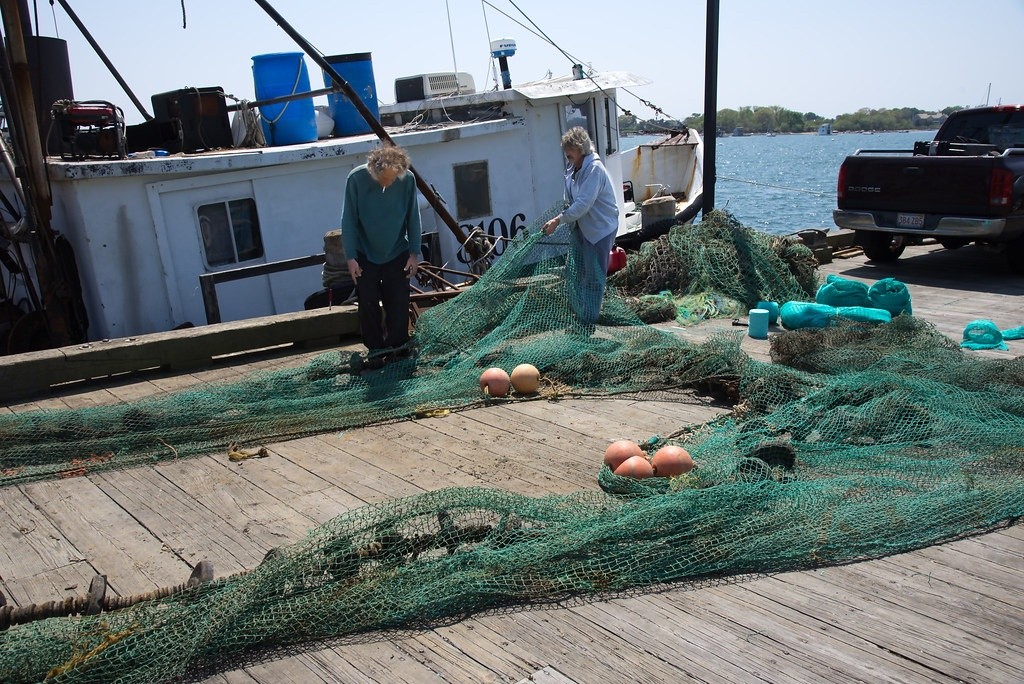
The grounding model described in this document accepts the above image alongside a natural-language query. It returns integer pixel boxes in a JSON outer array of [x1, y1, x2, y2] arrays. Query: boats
[[833, 128, 911, 136], [716, 126, 726, 137], [0, 0, 707, 389], [733, 127, 743, 136], [766, 133, 776, 137]]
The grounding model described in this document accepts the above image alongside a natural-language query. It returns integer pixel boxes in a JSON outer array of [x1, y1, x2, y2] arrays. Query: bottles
[[127, 151, 154, 158], [155, 150, 170, 156]]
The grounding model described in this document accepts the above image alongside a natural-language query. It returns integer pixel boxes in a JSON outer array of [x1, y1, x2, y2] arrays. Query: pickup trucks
[[832, 107, 1024, 281]]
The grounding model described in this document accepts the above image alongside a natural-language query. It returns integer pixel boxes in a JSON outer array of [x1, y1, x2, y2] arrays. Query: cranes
[[997, 97, 1002, 106], [982, 82, 991, 106]]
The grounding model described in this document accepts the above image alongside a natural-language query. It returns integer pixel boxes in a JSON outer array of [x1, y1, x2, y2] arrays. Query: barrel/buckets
[[250, 53, 319, 146], [321, 51, 382, 135]]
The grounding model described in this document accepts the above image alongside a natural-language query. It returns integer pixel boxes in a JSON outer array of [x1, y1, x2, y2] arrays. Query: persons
[[540, 125, 619, 342], [341, 146, 422, 364]]
